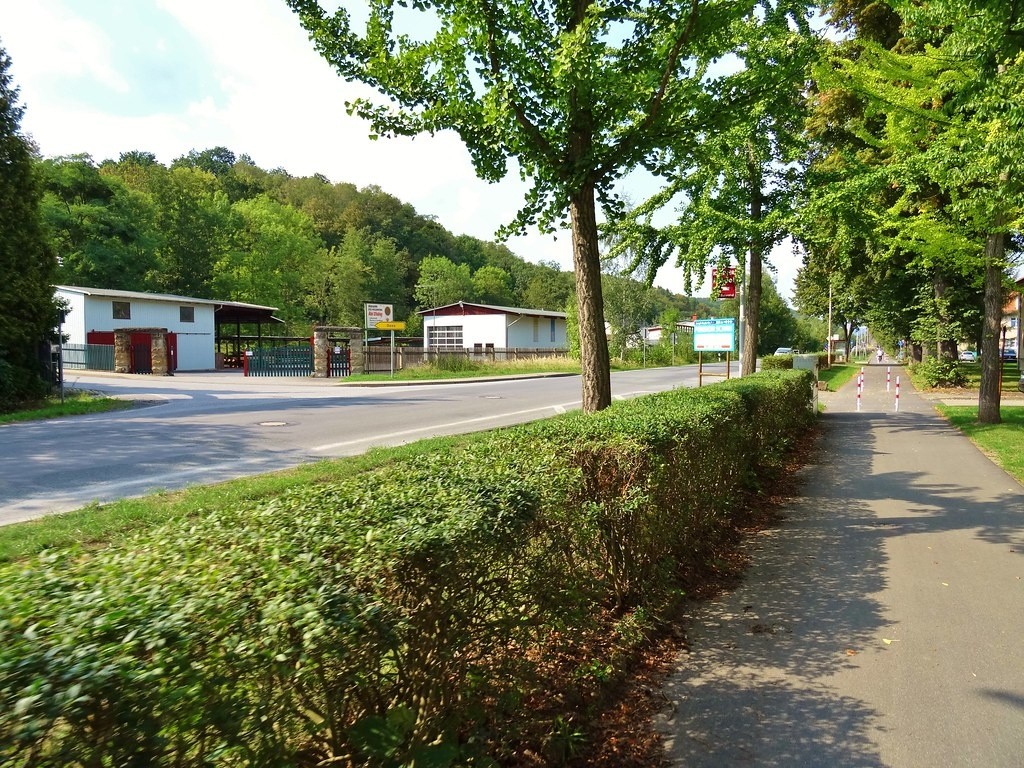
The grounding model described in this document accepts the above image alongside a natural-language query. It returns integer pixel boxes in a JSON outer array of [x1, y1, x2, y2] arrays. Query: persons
[[876, 348, 883, 361]]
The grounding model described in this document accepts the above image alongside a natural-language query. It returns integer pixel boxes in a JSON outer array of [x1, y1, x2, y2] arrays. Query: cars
[[773, 347, 791, 356], [999, 348, 1016, 358], [959, 350, 975, 363]]
